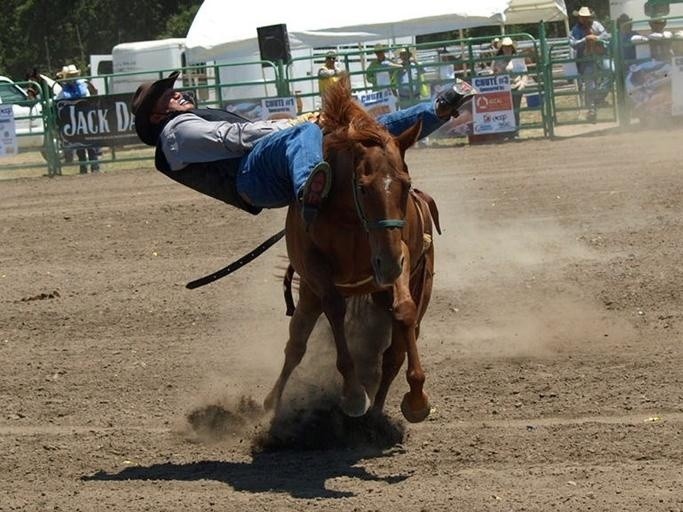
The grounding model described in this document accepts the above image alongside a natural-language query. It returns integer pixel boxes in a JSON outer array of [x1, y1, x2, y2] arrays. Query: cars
[[1, 75, 66, 164]]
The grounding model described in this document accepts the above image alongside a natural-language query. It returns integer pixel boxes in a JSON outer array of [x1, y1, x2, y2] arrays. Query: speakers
[[257, 24, 291, 67]]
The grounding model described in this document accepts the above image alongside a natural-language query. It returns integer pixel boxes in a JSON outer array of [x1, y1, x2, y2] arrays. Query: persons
[[567, 5, 618, 125], [316, 51, 344, 109], [128, 69, 480, 219], [364, 34, 532, 147], [614, 12, 639, 62], [649, 15, 674, 59], [51, 63, 106, 178]]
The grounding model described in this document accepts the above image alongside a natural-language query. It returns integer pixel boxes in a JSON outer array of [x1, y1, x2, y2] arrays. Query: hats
[[497, 37, 517, 48], [57, 64, 81, 76], [572, 7, 592, 17], [395, 48, 413, 56], [372, 44, 388, 52], [130, 71, 180, 145]]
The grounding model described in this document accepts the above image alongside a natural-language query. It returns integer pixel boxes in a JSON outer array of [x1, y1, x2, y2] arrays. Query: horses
[[435, 44, 479, 84], [508, 43, 542, 72], [263, 69, 435, 425]]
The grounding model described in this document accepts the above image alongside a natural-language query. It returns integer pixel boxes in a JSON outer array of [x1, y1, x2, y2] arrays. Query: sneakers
[[439, 77, 476, 107], [301, 162, 333, 226]]
